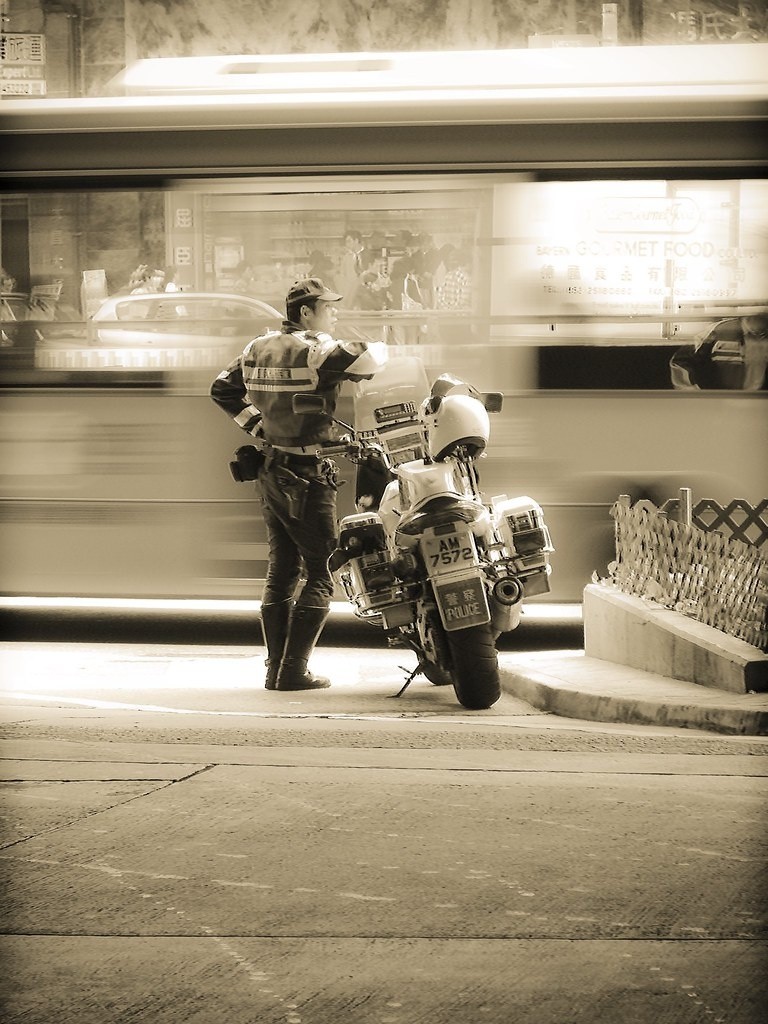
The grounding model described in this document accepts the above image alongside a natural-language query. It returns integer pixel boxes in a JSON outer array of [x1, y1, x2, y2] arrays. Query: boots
[[259, 596, 297, 688], [275, 604, 331, 689]]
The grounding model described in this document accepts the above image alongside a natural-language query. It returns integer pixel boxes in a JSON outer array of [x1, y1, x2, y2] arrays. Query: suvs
[[92, 291, 384, 376]]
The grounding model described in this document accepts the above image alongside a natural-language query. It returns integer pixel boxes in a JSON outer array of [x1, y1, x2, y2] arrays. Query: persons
[[1, 268, 26, 298], [134, 267, 191, 322], [209, 278, 376, 692], [299, 220, 472, 319], [670, 313, 768, 391], [218, 257, 291, 296]]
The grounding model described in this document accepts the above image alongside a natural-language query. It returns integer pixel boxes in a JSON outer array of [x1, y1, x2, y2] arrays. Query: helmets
[[417, 396, 489, 461]]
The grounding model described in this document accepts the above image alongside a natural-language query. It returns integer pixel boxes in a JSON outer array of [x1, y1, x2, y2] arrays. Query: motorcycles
[[291, 345, 554, 710]]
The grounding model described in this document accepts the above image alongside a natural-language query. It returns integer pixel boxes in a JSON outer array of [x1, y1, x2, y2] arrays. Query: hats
[[286, 277, 343, 308]]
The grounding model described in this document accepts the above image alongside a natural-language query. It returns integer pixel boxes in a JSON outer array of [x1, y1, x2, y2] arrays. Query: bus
[[0, 44, 768, 597]]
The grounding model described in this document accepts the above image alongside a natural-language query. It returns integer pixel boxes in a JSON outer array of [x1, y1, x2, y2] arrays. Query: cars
[[0, 293, 102, 367]]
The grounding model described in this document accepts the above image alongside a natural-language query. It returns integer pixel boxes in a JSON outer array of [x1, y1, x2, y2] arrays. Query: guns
[[273, 465, 311, 521]]
